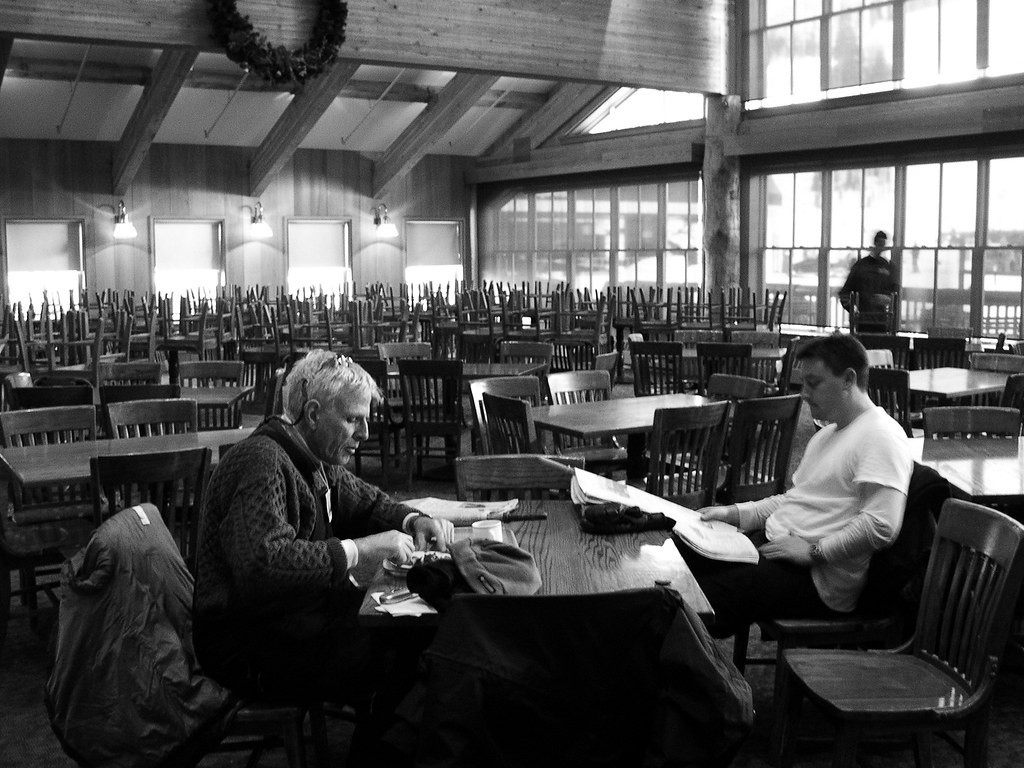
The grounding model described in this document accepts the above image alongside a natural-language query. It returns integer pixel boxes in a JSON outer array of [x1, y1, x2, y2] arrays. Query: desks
[[385, 363, 548, 378], [908, 437, 1024, 505], [92, 385, 255, 406], [906, 368, 1010, 406], [354, 499, 716, 630], [533, 392, 736, 480], [633, 346, 788, 379], [1, 428, 255, 486]]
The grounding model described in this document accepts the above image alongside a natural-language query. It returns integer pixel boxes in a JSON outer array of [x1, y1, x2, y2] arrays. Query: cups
[[471, 519, 502, 543]]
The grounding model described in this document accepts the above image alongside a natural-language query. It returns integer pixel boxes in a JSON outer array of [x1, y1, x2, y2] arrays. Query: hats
[[448, 538, 543, 595]]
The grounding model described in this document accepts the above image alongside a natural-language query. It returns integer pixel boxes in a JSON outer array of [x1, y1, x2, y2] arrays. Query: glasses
[[315, 354, 353, 375]]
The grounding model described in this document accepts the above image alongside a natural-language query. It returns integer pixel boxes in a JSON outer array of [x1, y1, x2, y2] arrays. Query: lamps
[[111, 198, 399, 240]]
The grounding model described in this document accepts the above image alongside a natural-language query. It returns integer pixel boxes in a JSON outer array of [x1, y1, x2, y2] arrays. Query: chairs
[[1, 279, 1024, 767]]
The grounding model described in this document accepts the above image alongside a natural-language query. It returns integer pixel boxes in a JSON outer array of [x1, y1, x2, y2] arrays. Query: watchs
[[808, 544, 825, 563]]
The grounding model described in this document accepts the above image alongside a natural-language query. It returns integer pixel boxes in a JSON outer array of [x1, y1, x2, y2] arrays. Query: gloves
[[578, 503, 676, 535]]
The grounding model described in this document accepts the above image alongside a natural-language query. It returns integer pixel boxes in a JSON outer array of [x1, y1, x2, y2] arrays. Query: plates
[[382, 550, 451, 577]]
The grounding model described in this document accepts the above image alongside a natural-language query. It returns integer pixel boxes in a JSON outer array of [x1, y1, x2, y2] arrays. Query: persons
[[191, 347, 454, 767], [837, 230, 899, 332], [673, 335, 914, 641]]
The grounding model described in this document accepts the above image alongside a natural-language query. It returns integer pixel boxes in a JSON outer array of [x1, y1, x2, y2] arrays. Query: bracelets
[[407, 512, 433, 538]]
